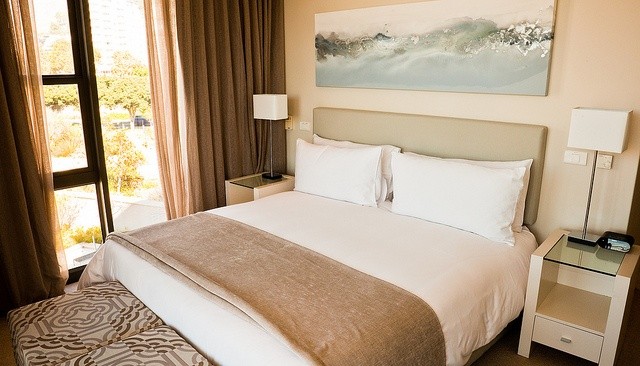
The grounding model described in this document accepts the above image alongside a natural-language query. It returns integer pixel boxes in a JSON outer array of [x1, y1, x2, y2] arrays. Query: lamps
[[252, 91, 289, 178], [566, 107, 632, 248]]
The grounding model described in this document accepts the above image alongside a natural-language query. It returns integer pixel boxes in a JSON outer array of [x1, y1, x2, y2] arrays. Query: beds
[[77, 108, 549, 366]]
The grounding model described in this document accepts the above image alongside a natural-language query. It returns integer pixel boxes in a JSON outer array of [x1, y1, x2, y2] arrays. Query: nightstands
[[517, 226, 640, 366], [225, 172, 294, 208]]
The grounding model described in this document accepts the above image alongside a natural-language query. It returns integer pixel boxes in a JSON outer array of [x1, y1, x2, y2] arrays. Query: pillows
[[294, 138, 385, 209], [401, 150, 533, 233], [312, 134, 401, 202], [389, 151, 526, 247]]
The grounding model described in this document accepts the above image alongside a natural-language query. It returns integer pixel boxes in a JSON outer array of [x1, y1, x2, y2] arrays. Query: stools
[[6, 282, 164, 366], [58, 325, 214, 366]]
